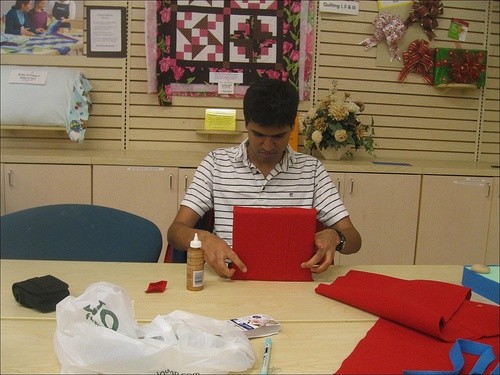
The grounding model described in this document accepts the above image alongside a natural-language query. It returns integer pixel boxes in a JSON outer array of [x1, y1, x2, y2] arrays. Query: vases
[[317, 146, 349, 161]]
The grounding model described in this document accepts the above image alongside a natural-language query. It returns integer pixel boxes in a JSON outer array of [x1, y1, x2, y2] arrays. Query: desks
[[0, 258, 500, 375]]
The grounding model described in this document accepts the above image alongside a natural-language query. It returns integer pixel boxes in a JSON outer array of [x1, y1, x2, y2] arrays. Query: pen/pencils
[[262, 338, 272, 372]]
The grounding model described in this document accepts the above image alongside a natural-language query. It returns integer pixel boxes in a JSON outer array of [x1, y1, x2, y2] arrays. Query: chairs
[[164, 206, 331, 266], [0, 204, 162, 262]]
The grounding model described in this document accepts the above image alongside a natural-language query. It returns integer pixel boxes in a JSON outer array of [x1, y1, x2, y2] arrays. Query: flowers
[[300, 79, 379, 164]]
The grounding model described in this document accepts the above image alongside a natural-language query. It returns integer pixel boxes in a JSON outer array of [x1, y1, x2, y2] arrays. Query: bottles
[[186, 233, 204, 291]]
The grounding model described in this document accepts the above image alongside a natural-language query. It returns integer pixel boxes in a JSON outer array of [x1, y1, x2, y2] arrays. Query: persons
[[5, 0, 45, 36], [27, 0, 49, 33], [48, 0, 76, 30], [167, 79, 362, 278]]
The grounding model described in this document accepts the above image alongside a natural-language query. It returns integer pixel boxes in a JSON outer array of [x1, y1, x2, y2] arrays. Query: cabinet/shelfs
[[0, 162, 91, 216], [320, 171, 421, 266], [415, 174, 500, 264], [93, 165, 198, 262]]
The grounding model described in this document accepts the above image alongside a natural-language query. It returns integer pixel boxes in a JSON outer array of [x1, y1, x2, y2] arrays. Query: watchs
[[329, 228, 347, 252]]
